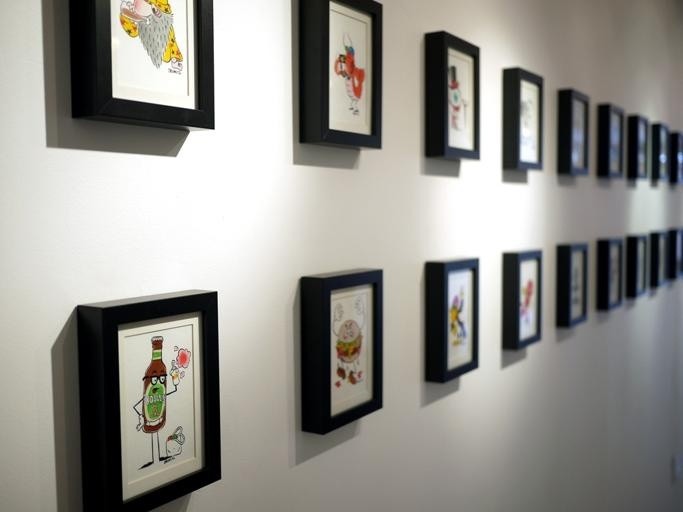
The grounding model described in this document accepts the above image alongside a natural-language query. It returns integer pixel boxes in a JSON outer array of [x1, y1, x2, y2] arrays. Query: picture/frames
[[596, 101, 683, 312], [424, 31, 480, 163], [500, 66, 545, 172], [68, 0, 215, 130], [556, 87, 590, 177], [298, 0, 383, 151], [555, 242, 589, 329], [502, 248, 543, 351], [300, 267, 384, 435], [74, 289, 221, 512], [423, 256, 479, 384]]
